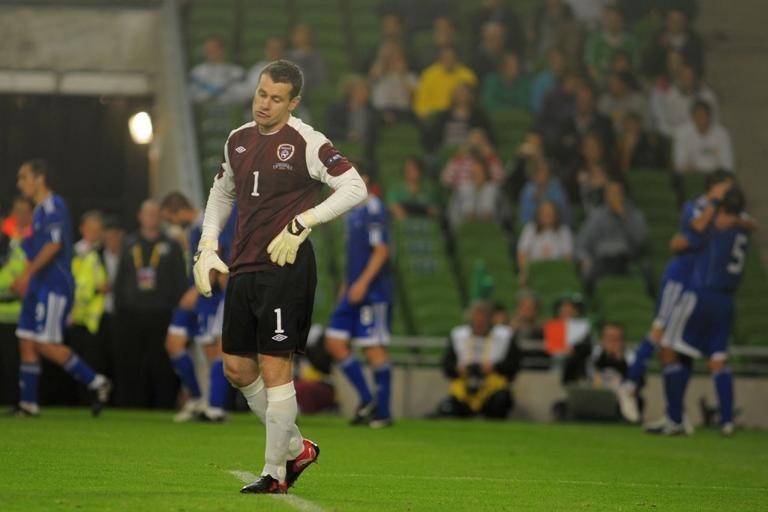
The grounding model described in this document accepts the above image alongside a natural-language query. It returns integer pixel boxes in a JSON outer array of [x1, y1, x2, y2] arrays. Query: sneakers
[[240, 474, 288, 494], [369, 411, 393, 428], [351, 399, 377, 425], [613, 380, 735, 440], [172, 400, 226, 422], [86, 375, 111, 417], [6, 402, 42, 419], [287, 438, 319, 489]]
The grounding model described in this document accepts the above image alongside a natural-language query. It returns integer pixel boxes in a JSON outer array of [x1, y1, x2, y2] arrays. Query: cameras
[[722, 187, 744, 215], [464, 360, 484, 396]]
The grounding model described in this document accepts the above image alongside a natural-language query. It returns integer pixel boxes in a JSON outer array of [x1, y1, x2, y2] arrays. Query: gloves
[[192, 250, 231, 299], [267, 215, 312, 267]]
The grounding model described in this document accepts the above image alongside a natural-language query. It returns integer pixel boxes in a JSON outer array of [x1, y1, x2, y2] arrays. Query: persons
[[325, 0, 735, 298], [429, 297, 645, 425], [189, 26, 330, 109], [615, 169, 758, 438], [191, 58, 371, 496], [322, 162, 394, 428], [0, 158, 229, 422]]
